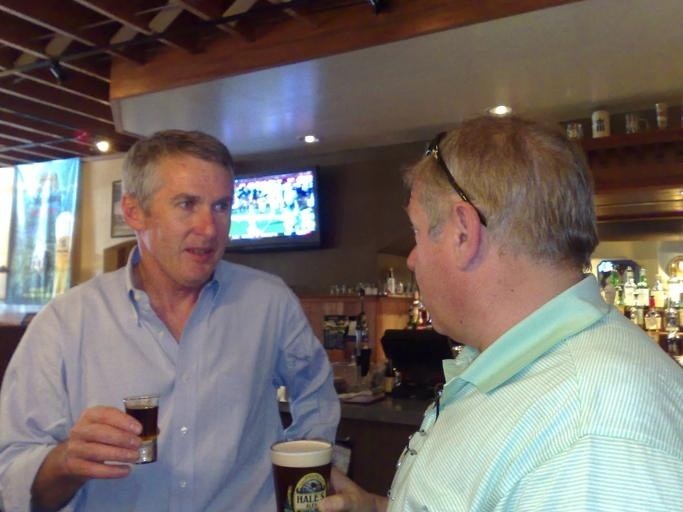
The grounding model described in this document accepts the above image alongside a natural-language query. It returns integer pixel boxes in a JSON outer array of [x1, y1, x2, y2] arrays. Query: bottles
[[381, 357, 396, 399], [408, 290, 423, 329], [386, 267, 395, 296], [591, 105, 610, 139], [565, 122, 584, 141], [604, 267, 682, 354]]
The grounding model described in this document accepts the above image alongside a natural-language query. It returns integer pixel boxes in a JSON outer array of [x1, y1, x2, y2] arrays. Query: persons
[[318, 116, 683, 512], [0, 130, 342, 512]]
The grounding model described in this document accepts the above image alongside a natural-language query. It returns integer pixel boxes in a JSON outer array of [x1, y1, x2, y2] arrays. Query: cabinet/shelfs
[[569, 124, 682, 240]]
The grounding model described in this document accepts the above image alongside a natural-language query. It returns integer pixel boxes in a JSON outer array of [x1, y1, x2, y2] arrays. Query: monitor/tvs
[[381, 328, 456, 394], [225, 164, 330, 253]]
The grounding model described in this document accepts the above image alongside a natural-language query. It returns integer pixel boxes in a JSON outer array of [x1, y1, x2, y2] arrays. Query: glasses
[[426, 131, 488, 227]]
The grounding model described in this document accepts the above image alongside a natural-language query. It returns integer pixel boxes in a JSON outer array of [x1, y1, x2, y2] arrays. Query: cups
[[656, 103, 668, 129], [625, 113, 648, 134], [120, 395, 158, 467], [327, 281, 379, 296], [268, 438, 333, 512], [330, 361, 356, 395]]
